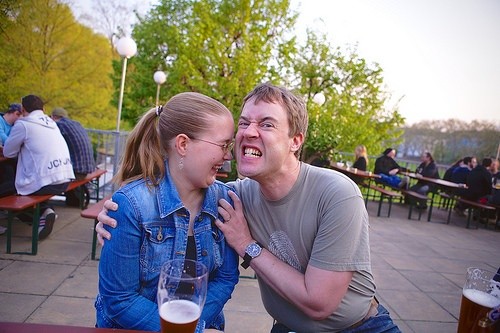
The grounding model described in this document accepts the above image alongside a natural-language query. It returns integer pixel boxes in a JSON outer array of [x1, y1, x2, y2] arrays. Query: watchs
[[240, 240, 266, 270]]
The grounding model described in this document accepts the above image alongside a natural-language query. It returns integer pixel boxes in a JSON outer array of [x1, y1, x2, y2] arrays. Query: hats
[[9, 103, 24, 113], [51, 108, 69, 119]]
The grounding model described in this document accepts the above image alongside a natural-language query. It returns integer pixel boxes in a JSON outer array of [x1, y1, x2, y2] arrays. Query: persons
[[304, 147, 322, 167], [0, 103, 23, 147], [349, 145, 369, 184], [50, 108, 96, 177], [438, 155, 500, 226], [3, 95, 75, 241], [93, 90, 241, 333], [95, 80, 402, 332], [410, 152, 440, 209], [374, 148, 410, 190]]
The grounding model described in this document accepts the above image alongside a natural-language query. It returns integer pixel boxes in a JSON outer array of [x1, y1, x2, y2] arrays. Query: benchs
[[0, 164, 114, 258], [360, 182, 496, 228]]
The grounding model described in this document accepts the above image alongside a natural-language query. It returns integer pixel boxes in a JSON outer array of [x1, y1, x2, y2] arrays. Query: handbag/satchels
[[67, 186, 91, 208]]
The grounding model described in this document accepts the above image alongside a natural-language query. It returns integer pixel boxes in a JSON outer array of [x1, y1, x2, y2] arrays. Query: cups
[[156, 259, 208, 333], [458, 267, 500, 333]]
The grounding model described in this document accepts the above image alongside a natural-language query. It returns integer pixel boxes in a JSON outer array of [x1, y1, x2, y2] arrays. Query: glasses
[[189, 137, 236, 152]]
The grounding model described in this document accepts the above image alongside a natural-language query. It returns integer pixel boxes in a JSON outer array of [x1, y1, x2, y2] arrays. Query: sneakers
[[37, 209, 55, 239]]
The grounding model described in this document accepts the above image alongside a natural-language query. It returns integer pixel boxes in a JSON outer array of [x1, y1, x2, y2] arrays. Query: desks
[[400, 170, 470, 222], [331, 162, 381, 187]]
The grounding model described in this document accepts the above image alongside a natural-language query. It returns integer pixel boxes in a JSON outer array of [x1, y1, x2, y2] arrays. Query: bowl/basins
[[337, 163, 344, 167]]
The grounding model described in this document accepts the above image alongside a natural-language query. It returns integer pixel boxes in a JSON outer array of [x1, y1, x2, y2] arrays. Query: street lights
[[153, 69, 168, 109], [114, 35, 139, 134]]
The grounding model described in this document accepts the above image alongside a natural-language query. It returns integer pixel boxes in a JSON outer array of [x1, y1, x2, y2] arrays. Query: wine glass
[[345, 161, 353, 170]]
